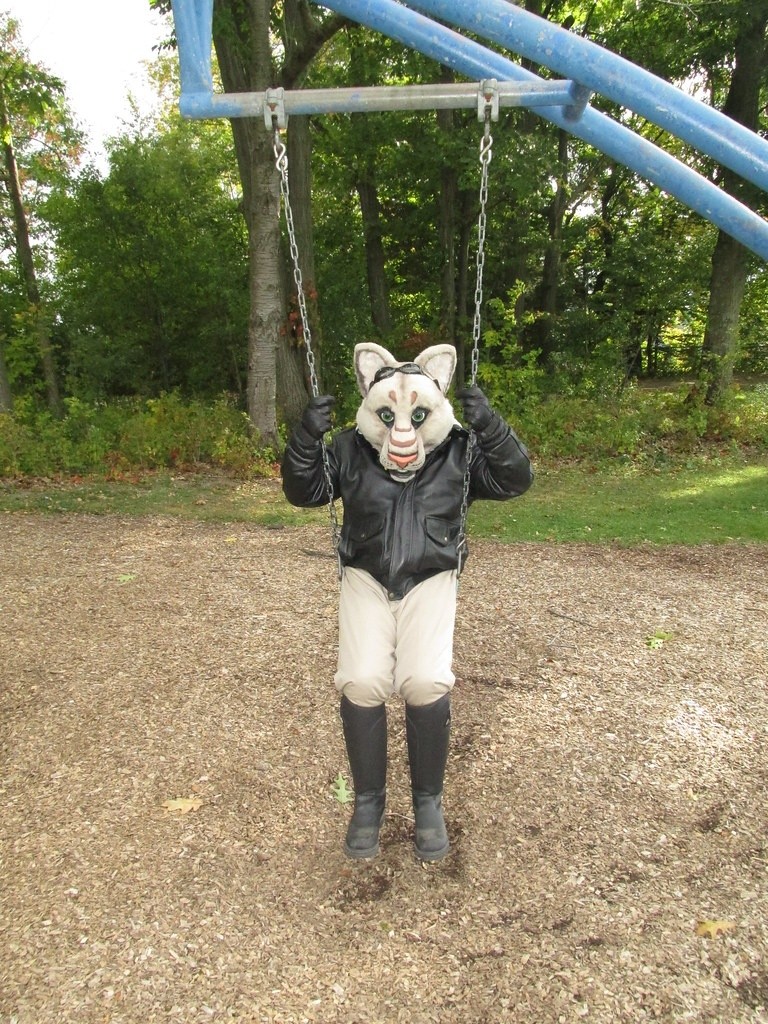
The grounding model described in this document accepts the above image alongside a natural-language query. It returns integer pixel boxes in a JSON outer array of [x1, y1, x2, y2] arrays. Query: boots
[[339, 695, 386, 856], [404, 692, 449, 858]]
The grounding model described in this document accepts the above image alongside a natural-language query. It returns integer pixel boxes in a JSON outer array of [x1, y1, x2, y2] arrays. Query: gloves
[[303, 394, 335, 438], [454, 386, 492, 431]]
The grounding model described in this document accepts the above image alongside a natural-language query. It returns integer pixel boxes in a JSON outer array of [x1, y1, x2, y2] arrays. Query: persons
[[281, 343, 535, 860]]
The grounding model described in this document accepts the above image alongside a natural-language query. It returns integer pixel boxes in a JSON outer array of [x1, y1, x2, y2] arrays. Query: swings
[[259, 82, 505, 580]]
